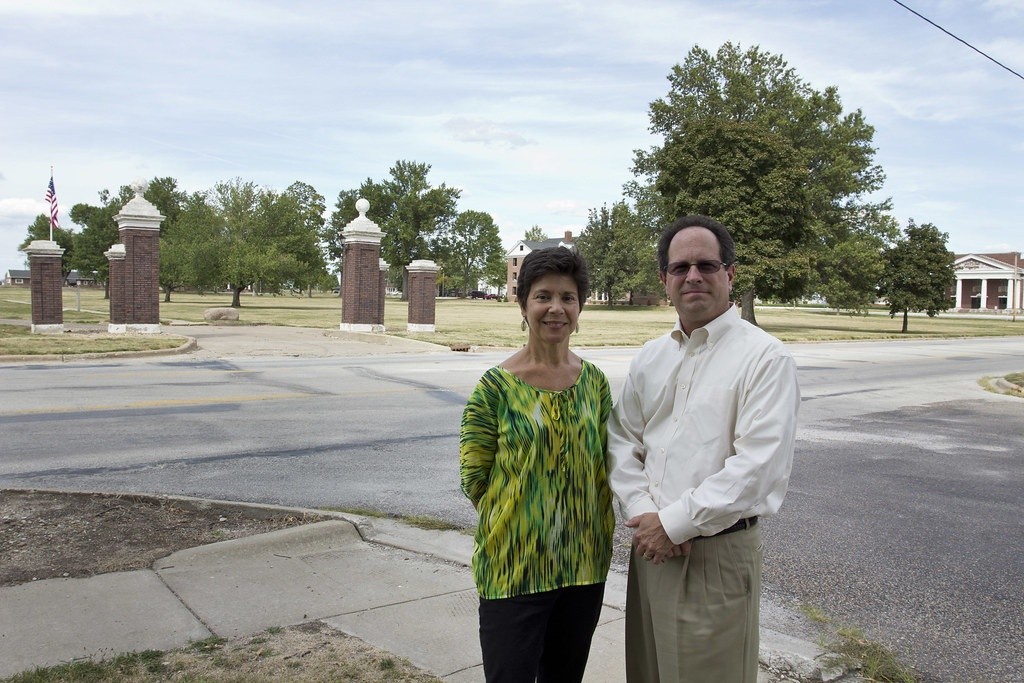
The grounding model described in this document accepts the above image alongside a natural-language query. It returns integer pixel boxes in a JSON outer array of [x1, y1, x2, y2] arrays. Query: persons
[[606, 215, 802, 682], [45, 176, 58, 230], [461, 244, 613, 682]]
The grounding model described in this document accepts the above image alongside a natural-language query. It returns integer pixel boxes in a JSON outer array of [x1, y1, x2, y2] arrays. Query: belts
[[693, 514, 758, 543]]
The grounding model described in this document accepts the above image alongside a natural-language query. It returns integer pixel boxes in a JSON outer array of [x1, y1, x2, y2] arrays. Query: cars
[[442, 287, 505, 300]]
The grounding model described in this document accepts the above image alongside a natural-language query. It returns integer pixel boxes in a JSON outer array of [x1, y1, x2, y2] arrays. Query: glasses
[[663, 258, 728, 276]]
